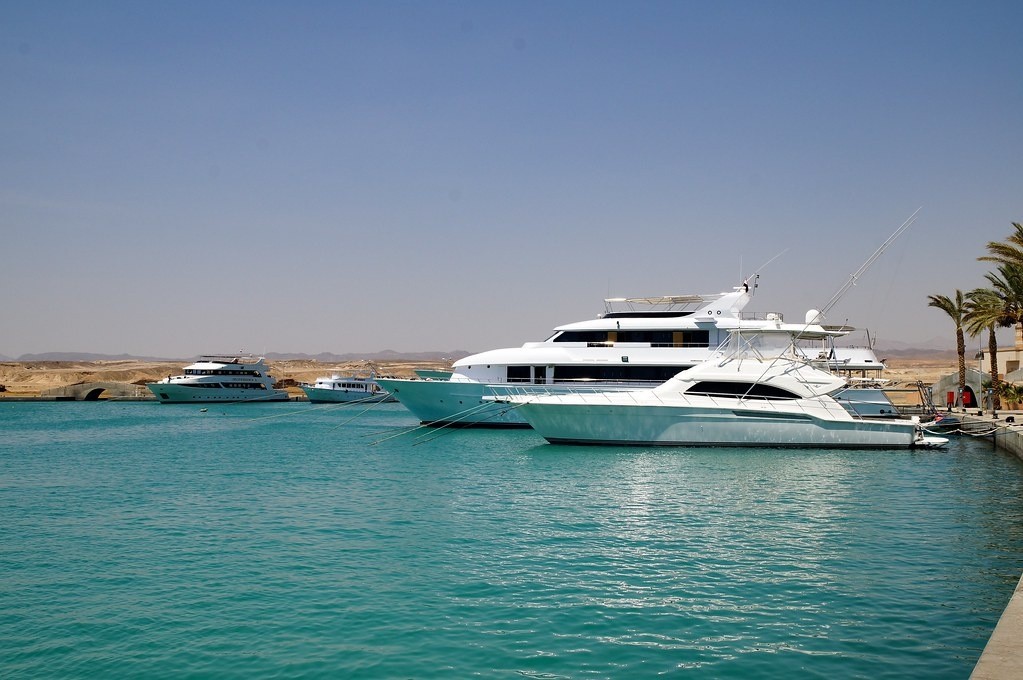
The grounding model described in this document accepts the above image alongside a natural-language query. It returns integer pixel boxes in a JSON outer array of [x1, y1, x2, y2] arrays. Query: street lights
[[442, 357, 451, 371], [278, 361, 288, 391], [361, 360, 371, 377]]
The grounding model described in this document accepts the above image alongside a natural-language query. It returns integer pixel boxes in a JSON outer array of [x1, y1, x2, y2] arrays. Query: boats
[[297, 370, 401, 404], [371, 205, 963, 449], [144, 356, 289, 404]]
